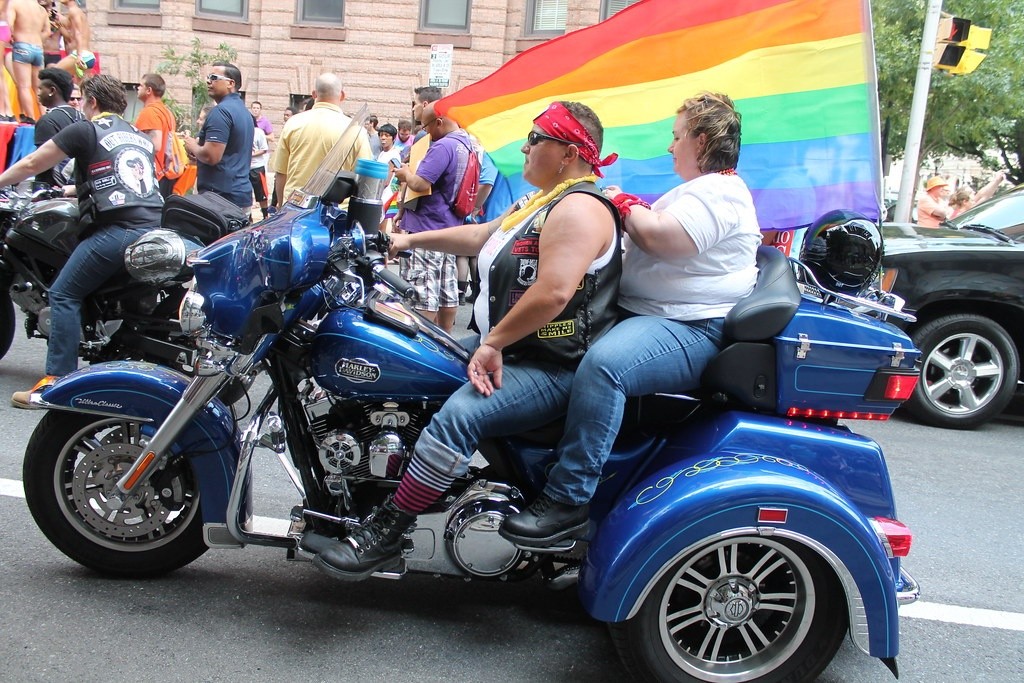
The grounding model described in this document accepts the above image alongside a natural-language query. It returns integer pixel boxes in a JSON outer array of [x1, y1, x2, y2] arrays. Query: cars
[[876, 183, 1024, 431]]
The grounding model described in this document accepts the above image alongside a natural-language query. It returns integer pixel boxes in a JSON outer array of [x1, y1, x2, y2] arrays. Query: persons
[[317, 101, 621, 582], [498, 89, 764, 541], [270, 73, 496, 336], [917, 174, 1006, 229], [0, 0, 95, 123], [0, 62, 273, 409]]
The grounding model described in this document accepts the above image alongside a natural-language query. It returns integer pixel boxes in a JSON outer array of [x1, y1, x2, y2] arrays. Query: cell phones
[[390, 158, 402, 168]]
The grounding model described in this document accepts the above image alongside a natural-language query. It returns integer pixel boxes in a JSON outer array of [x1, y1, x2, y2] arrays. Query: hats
[[925, 177, 947, 193]]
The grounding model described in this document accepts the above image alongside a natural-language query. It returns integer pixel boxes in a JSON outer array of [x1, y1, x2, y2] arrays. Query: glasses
[[206, 74, 233, 82], [527, 131, 583, 147], [422, 117, 442, 132], [68, 96, 82, 102]]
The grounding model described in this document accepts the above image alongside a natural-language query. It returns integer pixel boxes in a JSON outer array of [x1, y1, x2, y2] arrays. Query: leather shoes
[[498, 492, 591, 548]]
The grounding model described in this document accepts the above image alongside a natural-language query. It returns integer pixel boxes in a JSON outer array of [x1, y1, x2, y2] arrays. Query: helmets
[[799, 209, 883, 297]]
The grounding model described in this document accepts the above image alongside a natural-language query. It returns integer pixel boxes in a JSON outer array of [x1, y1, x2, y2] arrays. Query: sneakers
[[12, 377, 61, 409]]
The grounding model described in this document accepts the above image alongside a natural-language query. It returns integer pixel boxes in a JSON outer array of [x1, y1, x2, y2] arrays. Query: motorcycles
[[0, 181, 257, 406], [22, 102, 926, 682]]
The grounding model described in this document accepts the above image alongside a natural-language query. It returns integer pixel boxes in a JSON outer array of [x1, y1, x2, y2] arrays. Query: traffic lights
[[932, 16, 971, 70], [948, 24, 992, 76]]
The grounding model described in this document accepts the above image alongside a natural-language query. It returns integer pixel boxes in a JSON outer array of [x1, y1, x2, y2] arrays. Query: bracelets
[[610, 193, 651, 234]]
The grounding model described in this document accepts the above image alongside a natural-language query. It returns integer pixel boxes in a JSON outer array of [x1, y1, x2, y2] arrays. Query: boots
[[313, 494, 418, 582]]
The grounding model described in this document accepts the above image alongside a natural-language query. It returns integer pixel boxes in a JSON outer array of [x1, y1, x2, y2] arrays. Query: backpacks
[[147, 104, 188, 179], [439, 133, 480, 218]]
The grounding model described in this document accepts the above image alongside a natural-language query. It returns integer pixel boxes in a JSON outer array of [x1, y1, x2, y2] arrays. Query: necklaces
[[716, 167, 737, 176], [500, 174, 597, 231]]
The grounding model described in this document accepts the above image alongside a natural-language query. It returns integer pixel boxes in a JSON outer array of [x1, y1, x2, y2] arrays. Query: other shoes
[[0, 112, 37, 127]]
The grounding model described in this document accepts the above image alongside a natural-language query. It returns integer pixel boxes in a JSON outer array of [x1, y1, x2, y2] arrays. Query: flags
[[427, 0, 884, 232]]
